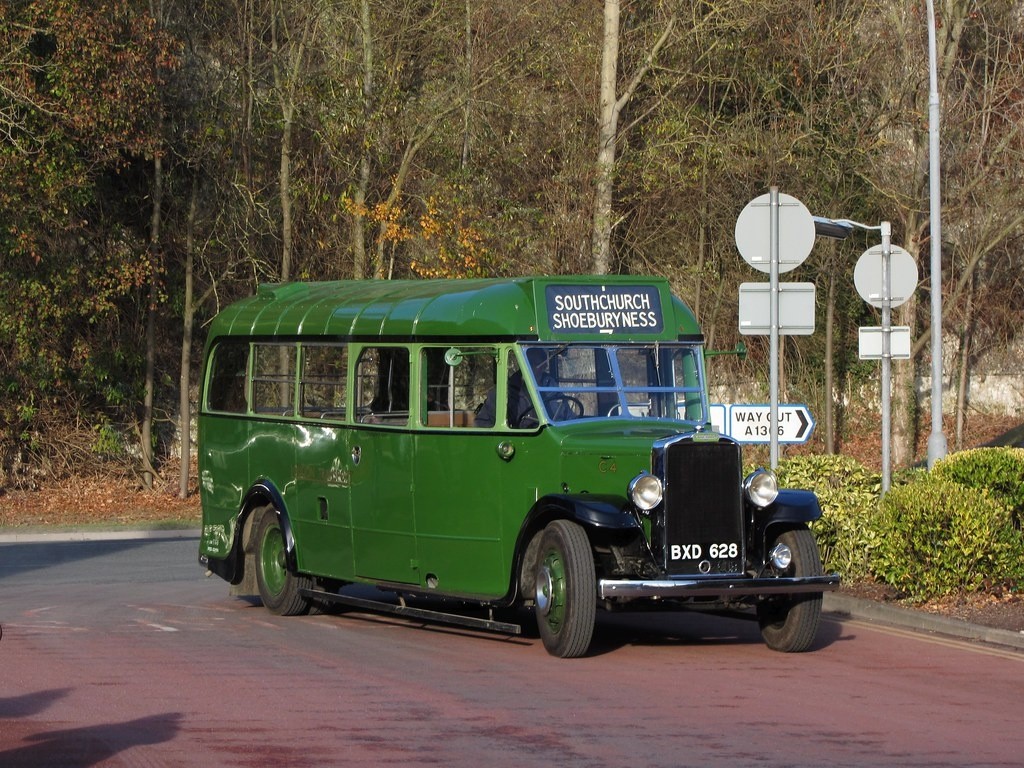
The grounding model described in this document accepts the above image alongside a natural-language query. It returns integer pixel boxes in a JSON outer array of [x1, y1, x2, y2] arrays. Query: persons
[[473, 349, 579, 428]]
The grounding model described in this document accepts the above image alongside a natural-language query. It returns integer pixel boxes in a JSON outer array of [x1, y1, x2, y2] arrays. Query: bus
[[197, 275, 843, 659]]
